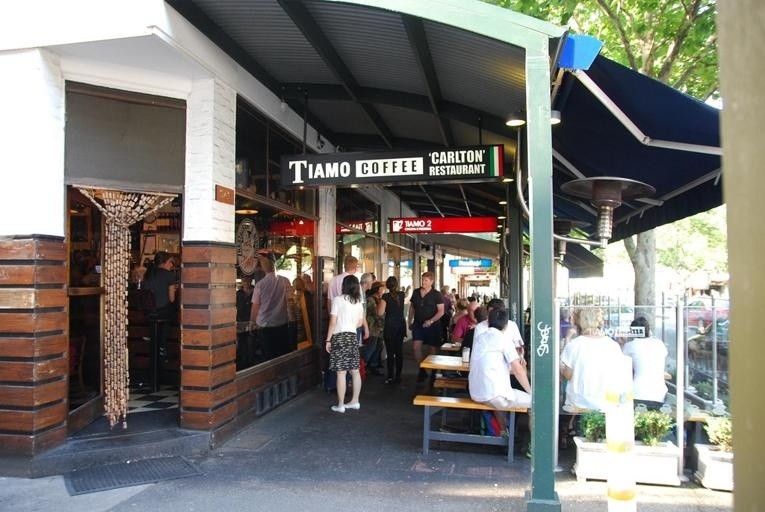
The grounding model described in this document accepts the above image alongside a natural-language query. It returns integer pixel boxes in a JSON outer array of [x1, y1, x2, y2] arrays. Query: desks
[[439, 342, 462, 351], [419, 353, 528, 377]]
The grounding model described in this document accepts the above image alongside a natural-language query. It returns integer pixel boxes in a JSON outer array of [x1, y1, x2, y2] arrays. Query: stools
[[144, 315, 173, 394]]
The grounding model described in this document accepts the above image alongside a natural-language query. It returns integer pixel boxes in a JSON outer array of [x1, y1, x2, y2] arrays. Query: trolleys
[[321, 328, 367, 393]]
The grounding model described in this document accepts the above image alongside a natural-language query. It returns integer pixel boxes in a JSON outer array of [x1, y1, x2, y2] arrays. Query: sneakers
[[331, 403, 360, 413]]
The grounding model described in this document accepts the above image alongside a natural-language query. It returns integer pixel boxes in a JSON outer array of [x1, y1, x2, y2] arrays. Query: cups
[[462, 347, 471, 362]]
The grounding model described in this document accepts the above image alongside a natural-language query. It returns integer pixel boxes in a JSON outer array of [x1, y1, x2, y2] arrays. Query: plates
[[455, 342, 462, 346]]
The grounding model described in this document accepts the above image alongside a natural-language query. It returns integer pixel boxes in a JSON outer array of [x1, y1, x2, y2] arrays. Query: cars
[[688, 312, 729, 398], [603, 299, 634, 321], [684, 298, 729, 337]]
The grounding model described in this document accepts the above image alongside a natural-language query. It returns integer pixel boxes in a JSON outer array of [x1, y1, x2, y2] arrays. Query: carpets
[[59, 452, 208, 499]]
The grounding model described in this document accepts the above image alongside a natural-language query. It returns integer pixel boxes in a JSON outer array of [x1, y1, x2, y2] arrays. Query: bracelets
[[324, 339, 334, 344]]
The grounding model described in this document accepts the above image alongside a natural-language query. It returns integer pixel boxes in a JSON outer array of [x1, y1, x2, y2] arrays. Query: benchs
[[560, 406, 708, 470], [412, 394, 528, 465], [433, 378, 481, 434]]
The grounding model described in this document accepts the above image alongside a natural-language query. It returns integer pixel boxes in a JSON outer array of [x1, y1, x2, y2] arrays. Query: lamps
[[506, 110, 525, 128], [550, 109, 561, 126]]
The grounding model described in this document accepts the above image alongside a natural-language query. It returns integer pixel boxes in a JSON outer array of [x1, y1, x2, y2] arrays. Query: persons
[[467, 307, 533, 459], [559, 303, 627, 415], [327, 255, 527, 412], [68, 244, 319, 393], [622, 317, 668, 410]]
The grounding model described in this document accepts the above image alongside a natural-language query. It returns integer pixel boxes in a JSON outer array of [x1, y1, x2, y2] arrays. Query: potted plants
[[692, 416, 735, 493], [570, 409, 683, 490]]
